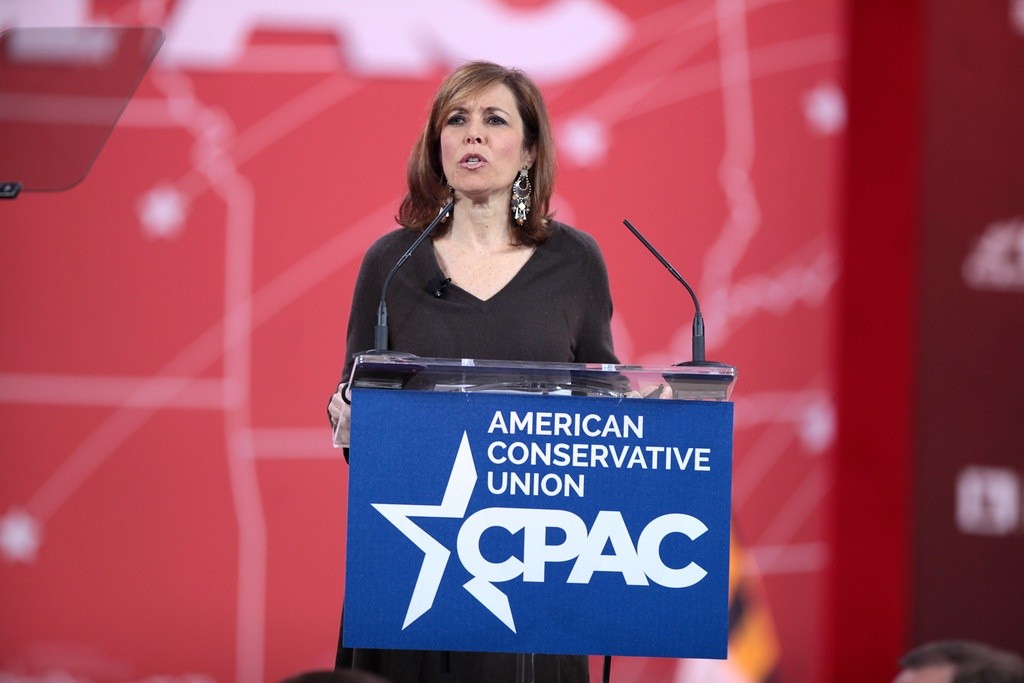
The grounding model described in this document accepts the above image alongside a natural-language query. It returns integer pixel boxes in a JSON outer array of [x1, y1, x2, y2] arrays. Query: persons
[[326, 60, 674, 683], [891, 638, 1024, 682]]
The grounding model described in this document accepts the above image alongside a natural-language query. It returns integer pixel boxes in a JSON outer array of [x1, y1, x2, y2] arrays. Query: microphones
[[623, 219, 737, 383], [348, 202, 454, 379], [428, 277, 443, 297]]
